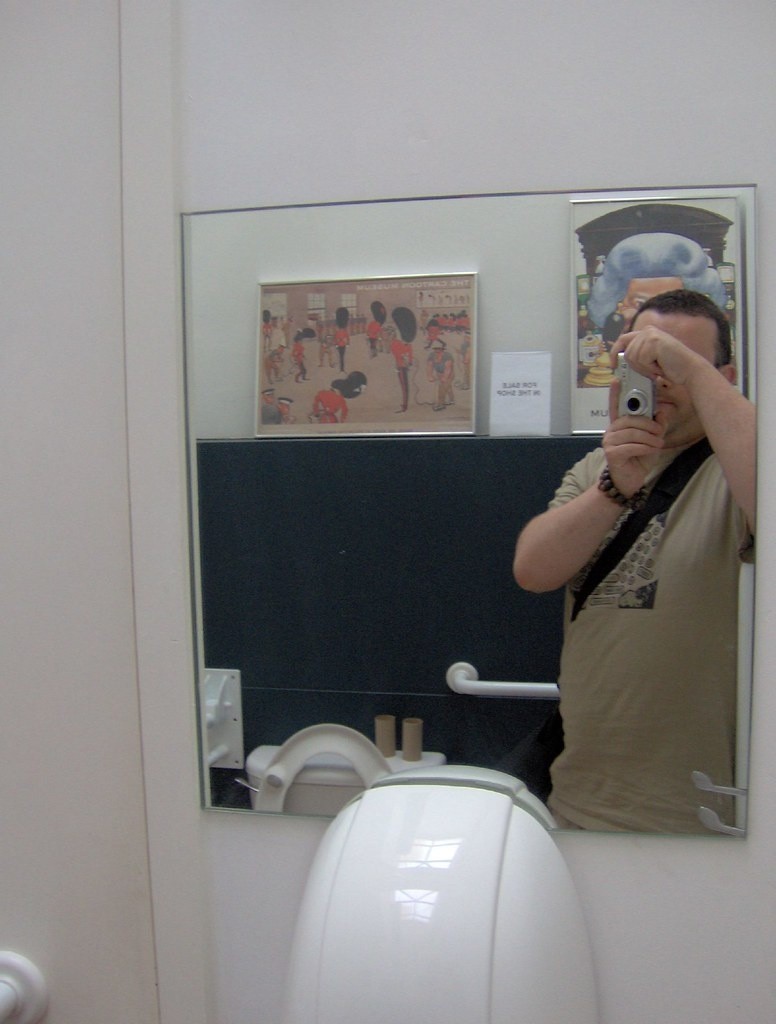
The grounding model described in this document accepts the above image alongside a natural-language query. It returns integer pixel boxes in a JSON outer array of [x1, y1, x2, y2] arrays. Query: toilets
[[247, 724, 446, 813]]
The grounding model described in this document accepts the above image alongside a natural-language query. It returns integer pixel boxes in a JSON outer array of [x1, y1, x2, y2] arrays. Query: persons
[[511, 290, 774, 835]]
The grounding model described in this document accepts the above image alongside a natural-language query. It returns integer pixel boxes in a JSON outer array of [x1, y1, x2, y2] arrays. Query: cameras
[[616, 353, 655, 423]]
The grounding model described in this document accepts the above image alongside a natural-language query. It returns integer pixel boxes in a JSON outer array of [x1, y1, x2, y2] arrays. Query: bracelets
[[598, 464, 649, 509]]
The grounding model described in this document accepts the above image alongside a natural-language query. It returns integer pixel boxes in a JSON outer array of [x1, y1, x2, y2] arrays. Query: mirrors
[[176, 181, 762, 843]]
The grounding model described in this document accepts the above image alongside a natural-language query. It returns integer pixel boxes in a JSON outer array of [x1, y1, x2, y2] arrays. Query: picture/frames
[[568, 196, 742, 435], [254, 272, 478, 438]]
